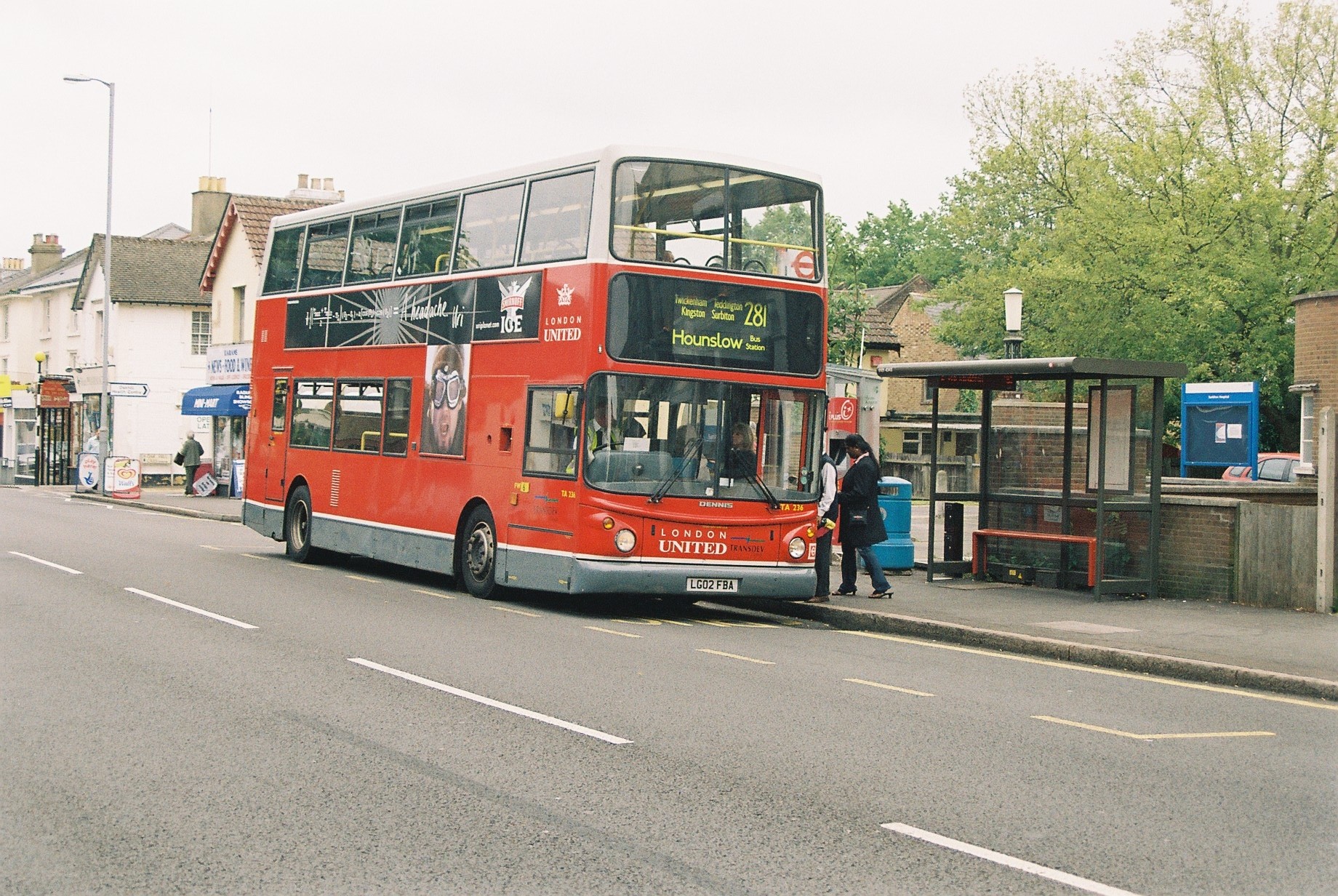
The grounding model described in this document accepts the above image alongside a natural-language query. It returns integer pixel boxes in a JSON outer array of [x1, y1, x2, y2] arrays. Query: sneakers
[[806, 595, 830, 603]]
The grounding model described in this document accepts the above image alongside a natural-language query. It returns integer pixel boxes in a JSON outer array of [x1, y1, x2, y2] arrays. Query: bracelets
[[108, 454, 109, 455]]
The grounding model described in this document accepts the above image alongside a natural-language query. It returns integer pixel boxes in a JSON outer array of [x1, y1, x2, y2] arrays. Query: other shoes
[[183, 493, 195, 497]]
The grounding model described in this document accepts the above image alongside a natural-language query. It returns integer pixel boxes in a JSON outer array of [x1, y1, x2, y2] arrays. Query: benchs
[[972, 528, 1104, 587]]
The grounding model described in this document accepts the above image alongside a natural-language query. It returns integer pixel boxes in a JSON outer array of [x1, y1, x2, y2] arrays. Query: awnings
[[181, 385, 251, 416]]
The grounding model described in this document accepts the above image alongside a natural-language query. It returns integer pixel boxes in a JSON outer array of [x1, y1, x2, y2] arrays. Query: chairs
[[744, 256, 768, 273], [705, 256, 725, 269], [274, 263, 394, 292], [674, 257, 691, 265]]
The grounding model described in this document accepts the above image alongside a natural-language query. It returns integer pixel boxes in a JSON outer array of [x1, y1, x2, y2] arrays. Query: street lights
[[34, 351, 46, 487], [63, 74, 114, 495]]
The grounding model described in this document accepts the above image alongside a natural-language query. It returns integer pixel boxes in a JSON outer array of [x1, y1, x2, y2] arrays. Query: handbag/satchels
[[174, 452, 185, 466], [849, 510, 868, 527]]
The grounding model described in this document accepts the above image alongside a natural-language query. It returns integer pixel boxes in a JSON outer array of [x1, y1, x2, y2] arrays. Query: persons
[[419, 344, 467, 457], [178, 430, 204, 494], [675, 423, 711, 482], [837, 434, 894, 600], [711, 420, 758, 486], [325, 403, 342, 444], [56, 452, 59, 460], [565, 396, 626, 476], [85, 428, 111, 490], [806, 450, 837, 602]]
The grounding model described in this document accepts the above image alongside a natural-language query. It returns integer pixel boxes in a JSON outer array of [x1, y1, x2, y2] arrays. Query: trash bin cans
[[862, 475, 915, 576]]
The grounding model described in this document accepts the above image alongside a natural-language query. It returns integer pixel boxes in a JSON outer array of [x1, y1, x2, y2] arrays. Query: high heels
[[832, 589, 857, 596], [868, 589, 894, 599]]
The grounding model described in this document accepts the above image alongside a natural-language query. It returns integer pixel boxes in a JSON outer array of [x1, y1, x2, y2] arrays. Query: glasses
[[433, 368, 465, 410]]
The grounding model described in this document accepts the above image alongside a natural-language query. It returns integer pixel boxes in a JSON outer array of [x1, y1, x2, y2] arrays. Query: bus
[[237, 151, 830, 606]]
